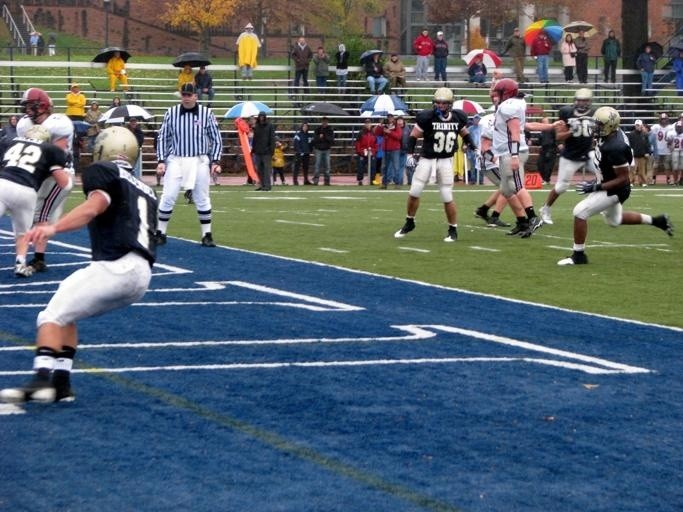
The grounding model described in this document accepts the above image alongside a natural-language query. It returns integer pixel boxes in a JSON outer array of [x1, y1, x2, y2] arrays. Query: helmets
[[591, 105, 621, 137], [659, 112, 683, 130], [24, 124, 51, 144], [489, 78, 519, 101], [91, 125, 141, 171], [431, 87, 454, 104], [19, 87, 51, 114], [573, 87, 594, 106]]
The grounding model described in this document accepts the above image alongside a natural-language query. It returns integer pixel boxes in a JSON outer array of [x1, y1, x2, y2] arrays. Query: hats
[[473, 114, 481, 119], [634, 119, 643, 126], [181, 83, 199, 95], [437, 31, 444, 35]]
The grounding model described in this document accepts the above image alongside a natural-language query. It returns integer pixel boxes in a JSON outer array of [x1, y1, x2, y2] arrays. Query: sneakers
[[504, 216, 544, 239], [443, 225, 458, 242], [242, 181, 400, 191], [0, 379, 57, 404], [630, 178, 683, 188], [155, 230, 167, 246], [538, 206, 554, 225], [557, 250, 589, 266], [52, 383, 76, 403], [394, 217, 417, 239], [656, 214, 675, 238], [201, 231, 217, 247], [416, 78, 447, 81], [14, 259, 33, 278], [473, 207, 512, 228], [27, 259, 50, 272]]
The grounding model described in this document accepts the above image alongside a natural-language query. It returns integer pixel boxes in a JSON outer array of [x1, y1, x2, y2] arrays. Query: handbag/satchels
[[570, 52, 579, 57]]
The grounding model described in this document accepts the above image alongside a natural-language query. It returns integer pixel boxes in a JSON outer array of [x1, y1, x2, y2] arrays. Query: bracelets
[[481, 150, 495, 161], [507, 142, 521, 157]]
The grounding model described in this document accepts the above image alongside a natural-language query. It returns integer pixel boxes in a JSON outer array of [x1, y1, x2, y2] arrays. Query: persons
[[391, 87, 480, 243], [246, 111, 335, 191], [357, 114, 482, 190], [411, 29, 489, 87], [0, 123, 159, 403], [176, 65, 214, 107], [235, 22, 262, 80], [504, 27, 623, 84], [66, 81, 145, 180], [473, 90, 565, 227], [489, 76, 543, 238], [291, 36, 407, 95], [1, 115, 21, 152], [537, 113, 682, 187], [13, 85, 77, 272], [537, 86, 598, 225], [1, 123, 74, 277], [635, 46, 681, 95], [107, 51, 128, 92], [147, 82, 223, 247], [556, 104, 674, 266], [30, 32, 57, 56]]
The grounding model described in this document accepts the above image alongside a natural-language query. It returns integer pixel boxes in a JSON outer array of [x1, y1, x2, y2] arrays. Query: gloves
[[405, 153, 420, 169], [474, 149, 485, 169], [574, 180, 602, 196]]
